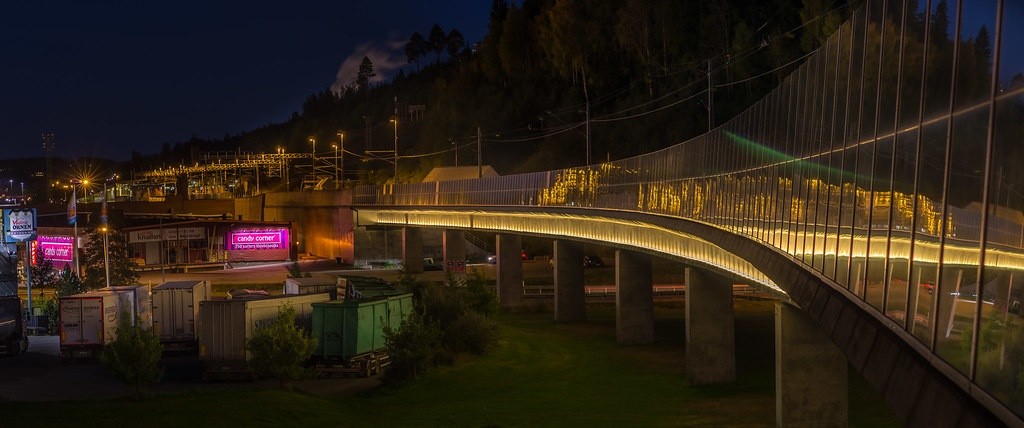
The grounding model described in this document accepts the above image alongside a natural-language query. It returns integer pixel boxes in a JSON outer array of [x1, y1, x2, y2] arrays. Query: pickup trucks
[[421, 258, 442, 270]]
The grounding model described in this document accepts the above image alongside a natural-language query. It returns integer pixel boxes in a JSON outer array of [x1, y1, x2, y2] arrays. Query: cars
[[487, 250, 528, 263], [584, 256, 605, 267]]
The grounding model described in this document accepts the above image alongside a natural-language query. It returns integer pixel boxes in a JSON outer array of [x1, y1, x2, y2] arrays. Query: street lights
[[84, 180, 87, 203], [332, 145, 338, 190], [389, 120, 397, 182], [337, 133, 343, 188], [310, 139, 315, 180]]
